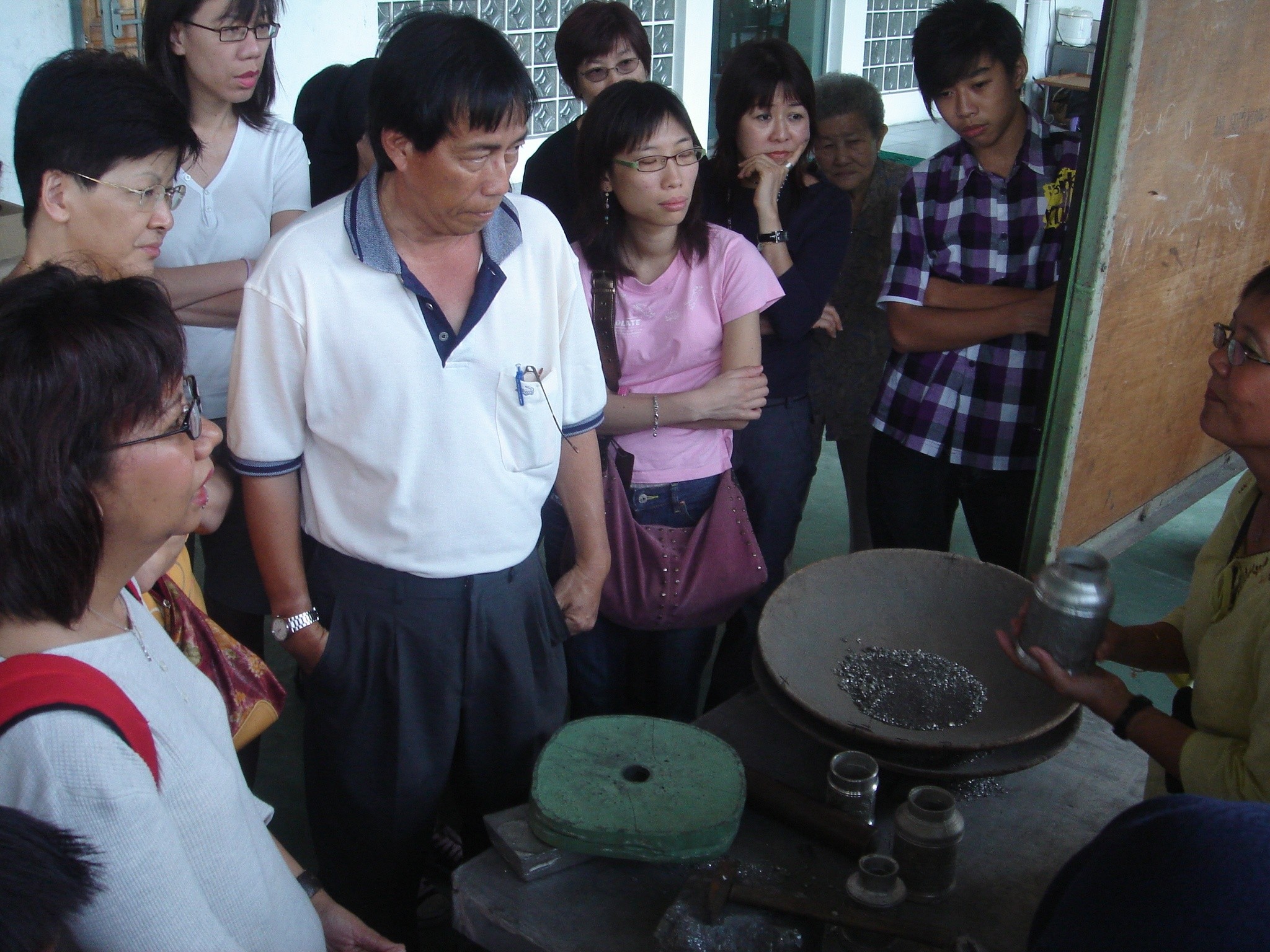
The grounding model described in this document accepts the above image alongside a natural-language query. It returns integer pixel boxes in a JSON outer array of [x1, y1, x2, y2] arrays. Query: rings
[[784, 161, 794, 170]]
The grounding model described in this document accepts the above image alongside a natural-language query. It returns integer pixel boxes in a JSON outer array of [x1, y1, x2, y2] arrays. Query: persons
[[995, 260, 1270, 952], [0, 0, 786, 649], [802, 72, 912, 552], [866, 0, 1085, 574], [700, 39, 850, 610], [0, 262, 406, 952]]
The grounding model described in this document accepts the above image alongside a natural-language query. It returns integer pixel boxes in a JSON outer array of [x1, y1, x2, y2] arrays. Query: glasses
[[577, 57, 640, 82], [60, 170, 188, 211], [1212, 323, 1270, 366], [609, 145, 706, 173], [185, 21, 281, 42], [99, 375, 203, 452]]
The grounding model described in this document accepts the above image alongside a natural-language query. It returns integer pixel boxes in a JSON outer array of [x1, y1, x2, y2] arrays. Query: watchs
[[272, 607, 320, 642], [757, 229, 788, 244]]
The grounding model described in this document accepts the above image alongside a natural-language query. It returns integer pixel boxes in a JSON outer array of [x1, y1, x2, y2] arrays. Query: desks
[[450, 684, 1149, 952]]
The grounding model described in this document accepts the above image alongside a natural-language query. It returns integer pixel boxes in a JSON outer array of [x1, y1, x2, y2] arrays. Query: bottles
[[1009, 545, 1113, 676], [826, 751, 879, 827], [893, 786, 964, 903], [840, 855, 905, 949]]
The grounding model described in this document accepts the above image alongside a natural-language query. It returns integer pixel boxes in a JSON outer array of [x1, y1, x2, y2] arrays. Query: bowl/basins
[[751, 548, 1082, 776]]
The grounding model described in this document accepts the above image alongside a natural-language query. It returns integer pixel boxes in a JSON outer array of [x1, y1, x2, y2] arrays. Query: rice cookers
[[1056, 6, 1093, 46]]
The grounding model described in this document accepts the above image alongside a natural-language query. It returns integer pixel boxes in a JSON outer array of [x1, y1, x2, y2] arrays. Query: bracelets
[[296, 870, 323, 897], [1112, 695, 1153, 740], [241, 257, 252, 279], [652, 396, 659, 437]]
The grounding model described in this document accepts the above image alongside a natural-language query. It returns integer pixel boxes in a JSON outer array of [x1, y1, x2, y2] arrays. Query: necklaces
[[86, 597, 151, 663], [1257, 522, 1268, 542]]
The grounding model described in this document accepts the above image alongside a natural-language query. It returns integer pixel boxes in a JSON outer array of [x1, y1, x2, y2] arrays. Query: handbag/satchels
[[553, 450, 768, 631], [153, 571, 287, 753]]
